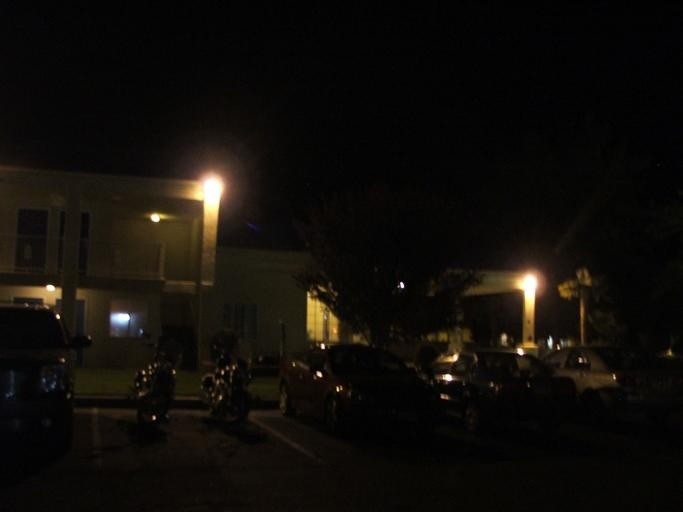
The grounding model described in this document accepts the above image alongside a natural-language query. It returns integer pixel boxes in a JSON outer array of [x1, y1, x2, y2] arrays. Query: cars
[[431, 348, 584, 433], [274, 343, 446, 438], [544, 343, 683, 423]]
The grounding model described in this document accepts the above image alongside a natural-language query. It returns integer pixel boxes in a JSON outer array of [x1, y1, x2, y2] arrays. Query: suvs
[[1, 295, 91, 450]]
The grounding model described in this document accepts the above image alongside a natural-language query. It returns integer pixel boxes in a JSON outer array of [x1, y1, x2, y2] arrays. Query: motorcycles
[[131, 354, 176, 431], [203, 343, 257, 426]]
